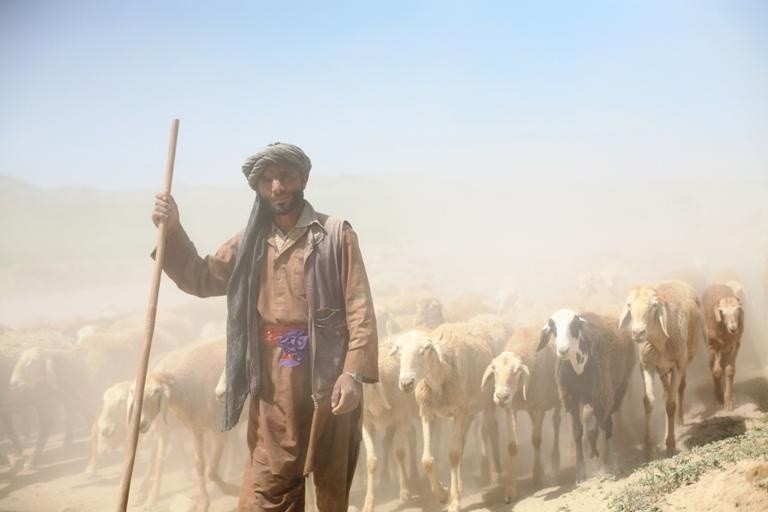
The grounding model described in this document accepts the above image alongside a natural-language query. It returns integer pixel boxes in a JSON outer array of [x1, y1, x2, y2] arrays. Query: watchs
[[344, 371, 364, 383]]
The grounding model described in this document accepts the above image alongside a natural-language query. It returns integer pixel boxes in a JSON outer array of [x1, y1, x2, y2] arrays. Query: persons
[[151, 138, 380, 512]]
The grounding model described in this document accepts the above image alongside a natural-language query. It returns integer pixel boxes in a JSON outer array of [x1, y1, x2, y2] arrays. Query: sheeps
[[0, 316, 246, 512], [361, 291, 556, 508], [536, 277, 700, 483], [703, 279, 748, 409]]
